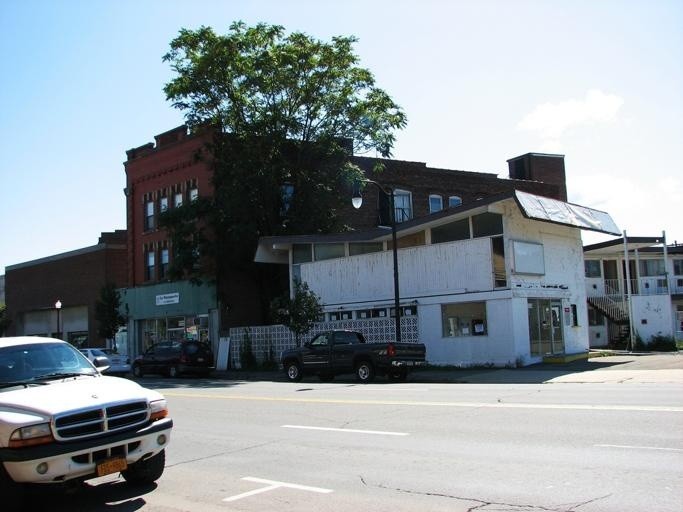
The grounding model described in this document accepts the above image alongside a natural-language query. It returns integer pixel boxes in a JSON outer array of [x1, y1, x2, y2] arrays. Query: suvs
[[131, 338, 215, 376], [0, 336, 173, 492]]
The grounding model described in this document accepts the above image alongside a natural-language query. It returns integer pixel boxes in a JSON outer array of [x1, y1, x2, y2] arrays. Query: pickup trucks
[[278, 327, 427, 384]]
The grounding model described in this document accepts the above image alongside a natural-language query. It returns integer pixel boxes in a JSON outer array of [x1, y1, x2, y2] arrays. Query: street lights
[[350, 176, 402, 347], [54, 299, 62, 338]]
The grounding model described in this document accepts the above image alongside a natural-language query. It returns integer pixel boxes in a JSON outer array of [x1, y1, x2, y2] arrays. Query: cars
[[98, 348, 132, 372], [56, 348, 110, 369]]
